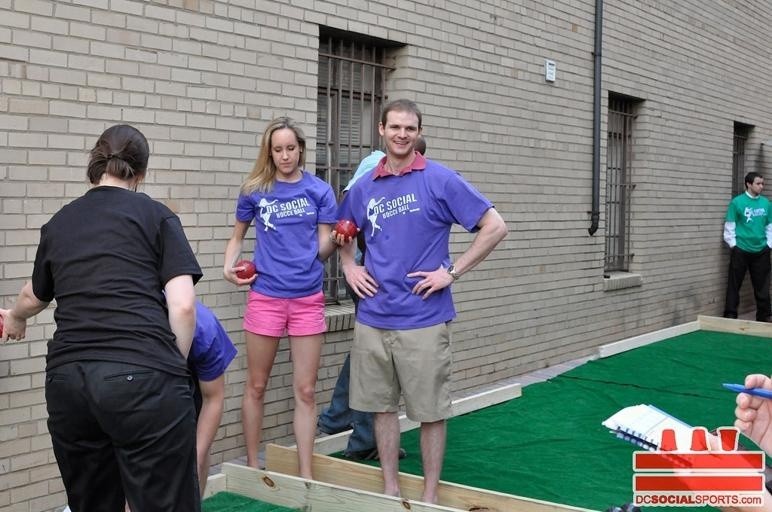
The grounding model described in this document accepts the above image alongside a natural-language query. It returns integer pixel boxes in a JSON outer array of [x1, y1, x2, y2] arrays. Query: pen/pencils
[[719, 383, 772, 399]]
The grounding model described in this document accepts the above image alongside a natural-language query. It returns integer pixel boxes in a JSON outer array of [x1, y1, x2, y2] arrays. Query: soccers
[[335, 220, 357, 242], [237, 260, 256, 280]]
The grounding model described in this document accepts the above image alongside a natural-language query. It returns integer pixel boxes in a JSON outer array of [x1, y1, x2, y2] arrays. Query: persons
[[158, 297, 238, 499], [221, 116, 359, 482], [0, 123, 207, 511], [730, 370, 771, 461], [722, 172, 772, 326], [311, 130, 429, 462], [333, 94, 509, 505]]
